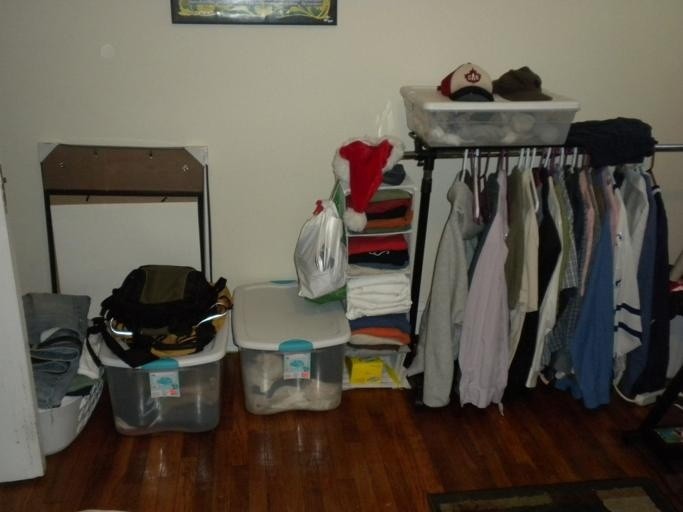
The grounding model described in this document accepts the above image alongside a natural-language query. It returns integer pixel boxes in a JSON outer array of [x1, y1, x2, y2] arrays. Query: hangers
[[442, 149, 668, 224]]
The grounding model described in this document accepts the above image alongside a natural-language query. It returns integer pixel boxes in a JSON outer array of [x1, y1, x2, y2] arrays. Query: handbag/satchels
[[99, 265, 219, 336]]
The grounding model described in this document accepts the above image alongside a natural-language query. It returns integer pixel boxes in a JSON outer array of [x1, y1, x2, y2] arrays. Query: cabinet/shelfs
[[333, 173, 419, 392]]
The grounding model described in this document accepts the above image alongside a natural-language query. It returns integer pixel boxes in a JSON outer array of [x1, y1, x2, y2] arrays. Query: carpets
[[425, 476, 678, 511]]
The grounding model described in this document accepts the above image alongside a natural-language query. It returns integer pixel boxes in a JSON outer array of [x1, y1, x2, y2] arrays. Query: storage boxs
[[400, 85, 579, 149], [99, 281, 352, 436]]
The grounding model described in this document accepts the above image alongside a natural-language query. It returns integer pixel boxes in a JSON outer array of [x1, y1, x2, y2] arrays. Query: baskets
[[38, 377, 103, 456]]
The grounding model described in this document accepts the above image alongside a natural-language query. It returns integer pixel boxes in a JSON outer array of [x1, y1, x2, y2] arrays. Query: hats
[[437, 62, 553, 102]]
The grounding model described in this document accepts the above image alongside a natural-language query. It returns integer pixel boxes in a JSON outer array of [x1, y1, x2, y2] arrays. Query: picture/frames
[[170, 0, 337, 26]]
